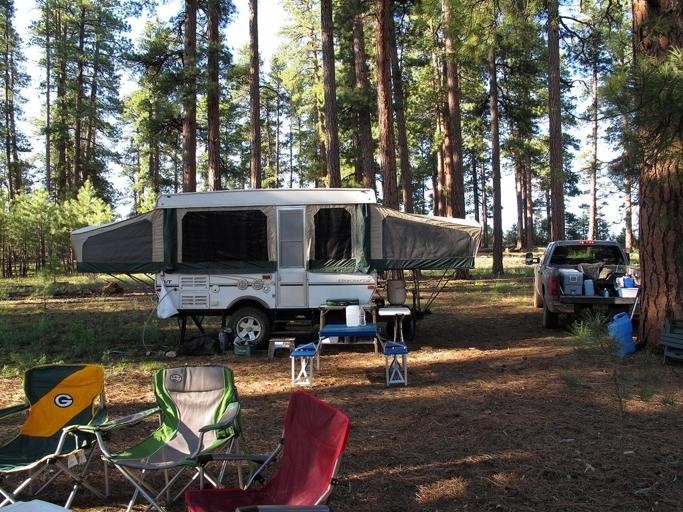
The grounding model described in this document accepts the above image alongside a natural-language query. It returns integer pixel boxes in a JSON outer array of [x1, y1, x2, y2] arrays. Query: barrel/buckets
[[387, 279, 407, 305], [623, 277, 635, 288], [607, 311, 636, 357]]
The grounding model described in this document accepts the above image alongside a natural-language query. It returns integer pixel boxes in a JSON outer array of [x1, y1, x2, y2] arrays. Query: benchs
[[381, 342, 406, 387], [287, 340, 320, 387]]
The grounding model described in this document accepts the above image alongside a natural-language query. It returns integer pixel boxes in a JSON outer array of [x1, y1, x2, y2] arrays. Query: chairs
[[0, 364, 109, 512], [178, 388, 347, 512], [91, 363, 246, 510], [657, 307, 682, 364]]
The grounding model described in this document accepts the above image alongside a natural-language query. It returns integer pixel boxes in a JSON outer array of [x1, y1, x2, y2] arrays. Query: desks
[[379, 305, 409, 341], [320, 325, 376, 352]]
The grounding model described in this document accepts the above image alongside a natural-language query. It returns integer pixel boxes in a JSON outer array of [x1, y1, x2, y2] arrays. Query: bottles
[[359, 306, 366, 326], [604, 288, 609, 298], [218, 329, 228, 351]]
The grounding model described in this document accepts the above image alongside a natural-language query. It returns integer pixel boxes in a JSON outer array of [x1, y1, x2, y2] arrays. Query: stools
[[266, 337, 295, 361]]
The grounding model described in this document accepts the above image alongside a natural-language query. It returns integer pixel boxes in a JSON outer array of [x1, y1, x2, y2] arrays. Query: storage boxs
[[618, 286, 637, 299], [559, 267, 581, 295]]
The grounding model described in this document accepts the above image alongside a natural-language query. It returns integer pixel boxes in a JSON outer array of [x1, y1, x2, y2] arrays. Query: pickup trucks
[[524, 238, 640, 327]]
